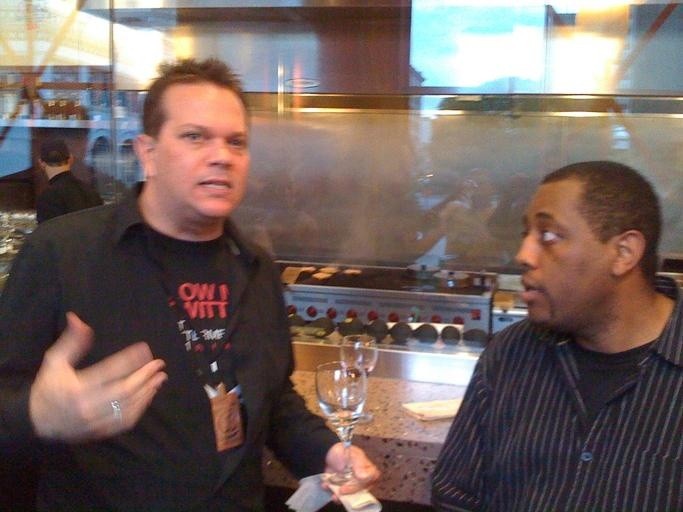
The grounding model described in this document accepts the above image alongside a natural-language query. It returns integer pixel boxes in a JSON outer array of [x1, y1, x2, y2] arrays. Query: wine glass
[[314, 361, 367, 485], [340, 334, 378, 424]]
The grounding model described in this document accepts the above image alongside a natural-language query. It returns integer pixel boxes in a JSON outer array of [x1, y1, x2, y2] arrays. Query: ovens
[[272, 260, 499, 341]]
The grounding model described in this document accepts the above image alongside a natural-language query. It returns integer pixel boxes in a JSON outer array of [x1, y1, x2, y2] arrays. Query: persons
[[34, 137, 105, 227], [425, 159, 683, 511], [0, 59, 382, 512], [1, 96, 127, 210]]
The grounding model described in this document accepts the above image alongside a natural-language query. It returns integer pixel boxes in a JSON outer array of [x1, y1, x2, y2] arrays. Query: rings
[[109, 398, 122, 418]]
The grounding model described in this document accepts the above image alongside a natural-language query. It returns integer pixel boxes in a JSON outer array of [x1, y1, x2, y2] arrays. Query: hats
[[40, 139, 69, 163]]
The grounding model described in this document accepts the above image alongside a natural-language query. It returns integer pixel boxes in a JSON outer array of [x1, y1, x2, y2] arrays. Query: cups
[[0, 210, 37, 255]]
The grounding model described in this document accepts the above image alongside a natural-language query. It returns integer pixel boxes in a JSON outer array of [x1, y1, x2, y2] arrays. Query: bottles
[[1, 84, 137, 122]]
